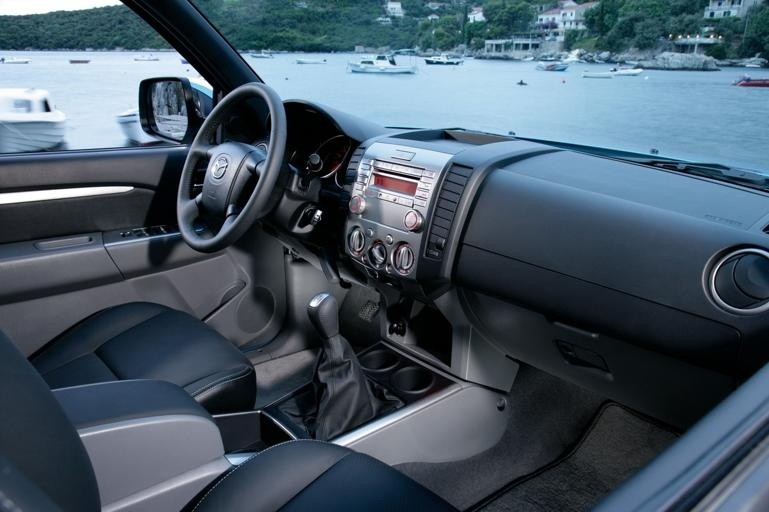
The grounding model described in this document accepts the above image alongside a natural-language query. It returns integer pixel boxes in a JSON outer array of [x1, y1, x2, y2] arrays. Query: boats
[[251, 53, 273, 58], [534, 60, 644, 79], [0, 86, 69, 155], [295, 57, 328, 65], [347, 53, 417, 74], [424, 55, 463, 65], [134, 57, 159, 61], [0, 56, 33, 64], [734, 75, 769, 87], [68, 59, 89, 64], [115, 107, 164, 147]]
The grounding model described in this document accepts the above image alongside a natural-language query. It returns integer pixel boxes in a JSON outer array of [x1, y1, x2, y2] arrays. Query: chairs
[[2, 333, 457, 510], [29, 301, 257, 418]]
